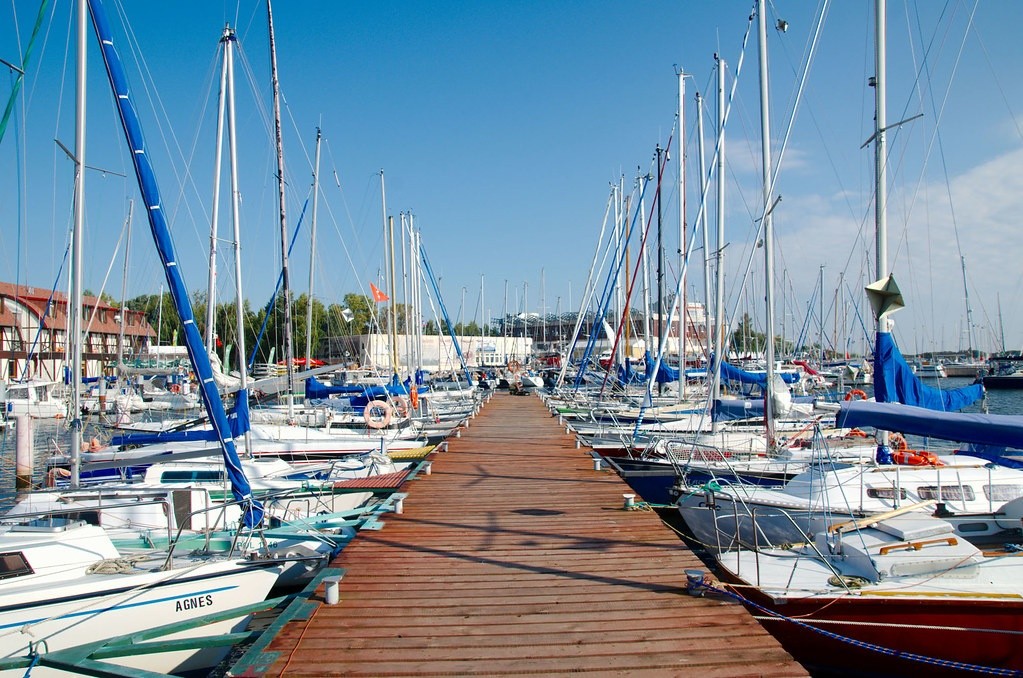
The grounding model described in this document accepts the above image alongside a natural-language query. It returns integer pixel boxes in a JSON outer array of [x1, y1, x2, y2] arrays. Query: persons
[[509, 382, 522, 395], [988, 358, 997, 376], [188, 371, 195, 384], [695, 356, 701, 367]]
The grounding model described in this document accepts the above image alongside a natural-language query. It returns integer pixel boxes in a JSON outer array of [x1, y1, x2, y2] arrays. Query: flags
[[371, 284, 388, 301]]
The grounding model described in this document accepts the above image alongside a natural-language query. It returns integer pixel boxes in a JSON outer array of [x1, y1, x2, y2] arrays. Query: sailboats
[[532, 0, 1023, 561], [0, 0, 491, 676], [432, 273, 545, 390]]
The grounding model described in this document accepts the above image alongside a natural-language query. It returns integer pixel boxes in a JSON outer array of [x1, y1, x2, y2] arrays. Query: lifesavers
[[888, 433, 907, 449], [507, 360, 520, 373], [892, 448, 939, 466], [845, 429, 867, 438], [410, 386, 419, 410], [386, 396, 409, 418], [845, 390, 867, 401], [363, 400, 392, 430]]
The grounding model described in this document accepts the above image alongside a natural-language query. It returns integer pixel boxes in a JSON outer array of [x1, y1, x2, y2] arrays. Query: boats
[[704, 400, 1023, 677]]
